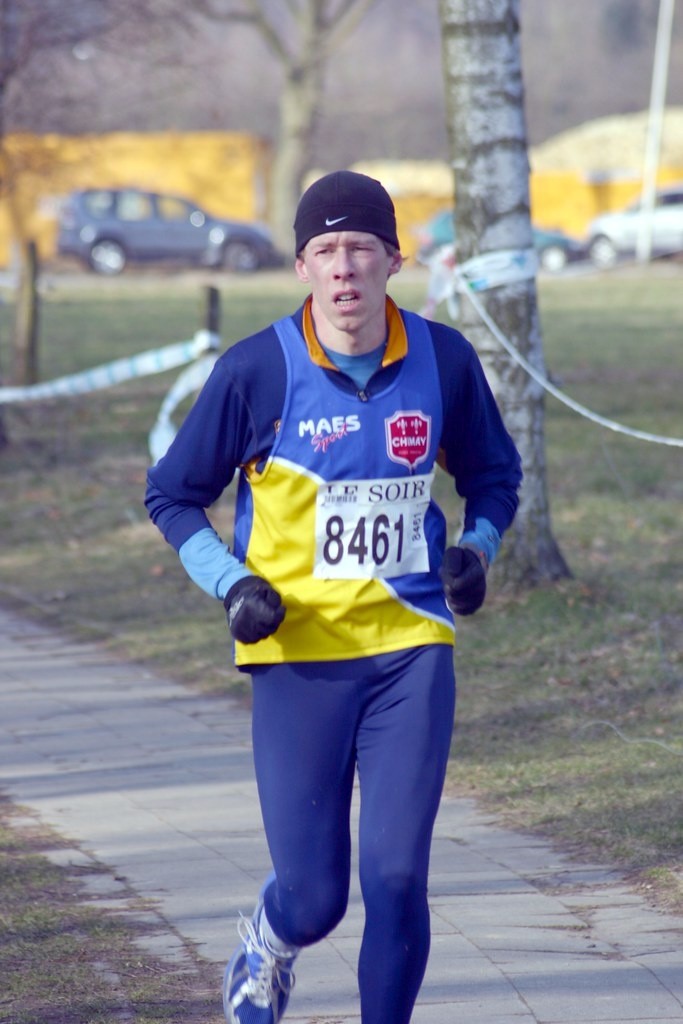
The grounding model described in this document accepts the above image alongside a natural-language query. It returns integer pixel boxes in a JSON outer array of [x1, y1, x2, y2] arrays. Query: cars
[[588, 190, 683, 270], [54, 186, 280, 278], [412, 211, 590, 273]]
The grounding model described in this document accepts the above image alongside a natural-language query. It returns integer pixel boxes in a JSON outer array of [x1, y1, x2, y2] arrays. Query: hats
[[293, 170, 400, 257]]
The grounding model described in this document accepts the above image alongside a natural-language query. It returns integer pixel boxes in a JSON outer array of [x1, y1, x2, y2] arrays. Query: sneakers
[[221, 868, 299, 1024]]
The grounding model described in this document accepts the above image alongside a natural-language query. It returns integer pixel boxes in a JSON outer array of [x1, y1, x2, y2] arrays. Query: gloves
[[438, 546, 487, 616], [222, 575, 287, 644]]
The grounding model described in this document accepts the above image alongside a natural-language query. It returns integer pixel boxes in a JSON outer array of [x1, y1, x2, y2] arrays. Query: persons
[[144, 170, 523, 1024]]
